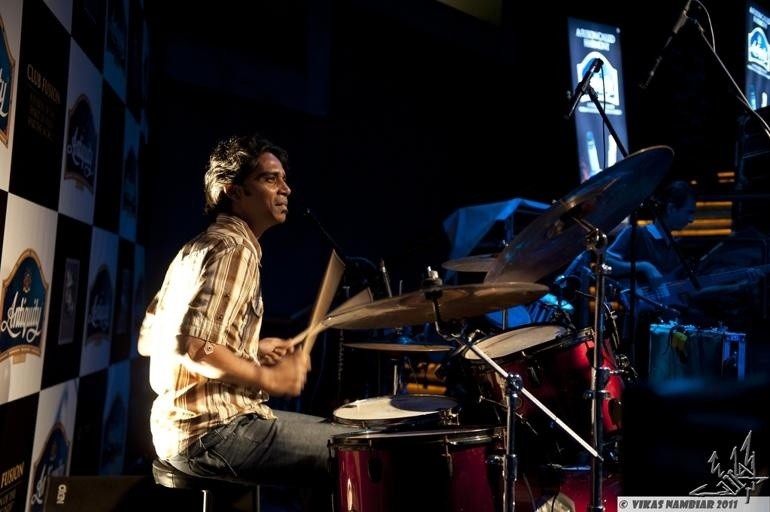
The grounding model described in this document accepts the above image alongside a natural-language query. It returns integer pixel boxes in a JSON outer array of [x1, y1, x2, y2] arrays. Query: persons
[[63, 270, 74, 313], [11, 269, 38, 308], [136, 132, 362, 485], [606, 180, 695, 301]]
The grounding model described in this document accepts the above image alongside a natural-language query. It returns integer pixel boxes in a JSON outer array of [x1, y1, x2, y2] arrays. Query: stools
[[151, 457, 263, 512]]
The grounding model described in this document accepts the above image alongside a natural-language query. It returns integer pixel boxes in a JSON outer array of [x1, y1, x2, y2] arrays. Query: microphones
[[564, 59, 599, 120], [638, 2, 690, 89], [302, 207, 346, 259]]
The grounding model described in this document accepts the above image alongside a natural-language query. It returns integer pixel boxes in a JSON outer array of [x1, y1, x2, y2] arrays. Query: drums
[[334, 395, 456, 421], [326, 428, 506, 511], [464, 324, 622, 450]]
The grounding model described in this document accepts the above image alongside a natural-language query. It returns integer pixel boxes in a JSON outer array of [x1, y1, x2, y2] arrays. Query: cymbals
[[485, 146, 673, 285], [444, 255, 499, 273], [324, 282, 549, 328], [344, 343, 454, 351]]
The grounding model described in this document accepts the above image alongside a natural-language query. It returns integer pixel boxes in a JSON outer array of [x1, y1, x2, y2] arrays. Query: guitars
[[621, 262, 769, 319]]
